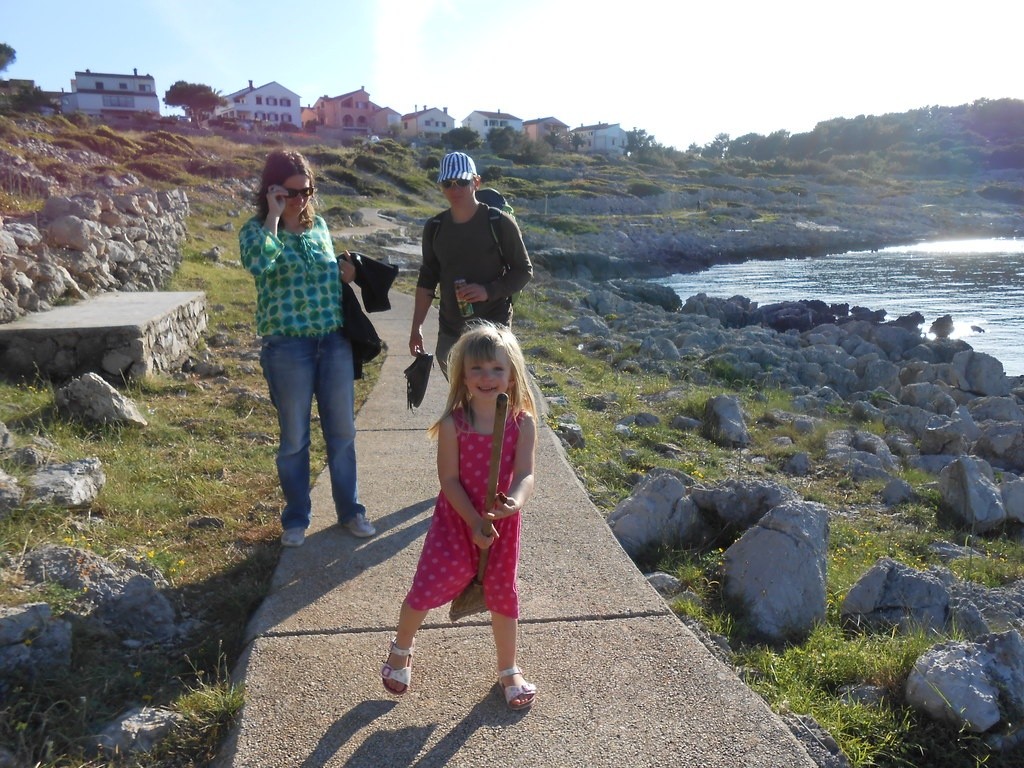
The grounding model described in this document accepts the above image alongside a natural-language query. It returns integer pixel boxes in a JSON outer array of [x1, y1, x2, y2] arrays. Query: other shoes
[[403, 351, 434, 410], [337, 510, 376, 537], [281, 527, 305, 546]]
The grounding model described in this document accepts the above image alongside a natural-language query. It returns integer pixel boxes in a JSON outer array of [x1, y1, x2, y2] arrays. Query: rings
[[271, 188, 276, 191]]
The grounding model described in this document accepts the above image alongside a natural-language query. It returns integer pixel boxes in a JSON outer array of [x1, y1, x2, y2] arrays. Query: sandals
[[380, 636, 415, 694], [498, 667, 536, 710]]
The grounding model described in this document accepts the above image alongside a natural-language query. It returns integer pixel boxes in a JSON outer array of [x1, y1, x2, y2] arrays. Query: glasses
[[442, 180, 470, 189], [279, 184, 313, 198]]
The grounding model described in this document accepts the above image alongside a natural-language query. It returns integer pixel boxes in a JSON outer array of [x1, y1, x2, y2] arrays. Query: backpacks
[[429, 187, 523, 306]]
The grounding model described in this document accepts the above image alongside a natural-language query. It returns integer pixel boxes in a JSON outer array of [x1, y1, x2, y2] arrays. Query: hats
[[437, 152, 477, 183]]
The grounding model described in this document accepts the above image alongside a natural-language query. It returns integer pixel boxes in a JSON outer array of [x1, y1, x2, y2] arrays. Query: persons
[[409, 150, 534, 382], [237, 148, 377, 547], [381, 317, 539, 712]]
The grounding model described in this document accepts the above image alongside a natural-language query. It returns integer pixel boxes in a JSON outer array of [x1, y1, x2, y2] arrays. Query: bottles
[[454, 278, 474, 317]]
